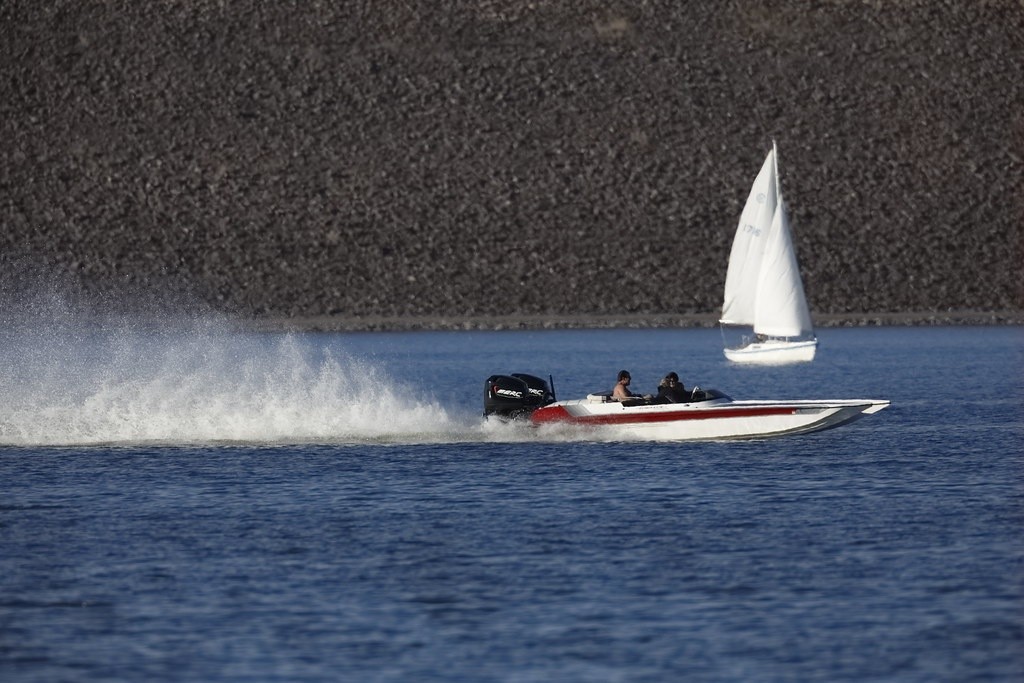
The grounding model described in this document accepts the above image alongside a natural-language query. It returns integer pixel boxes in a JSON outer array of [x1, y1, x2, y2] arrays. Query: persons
[[612, 369, 639, 401]]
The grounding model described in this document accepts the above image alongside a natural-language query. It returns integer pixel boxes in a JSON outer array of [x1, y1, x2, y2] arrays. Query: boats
[[716, 135, 818, 365], [477, 369, 890, 444]]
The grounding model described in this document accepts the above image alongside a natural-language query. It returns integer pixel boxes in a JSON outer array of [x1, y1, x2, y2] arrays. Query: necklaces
[[657, 371, 692, 402]]
